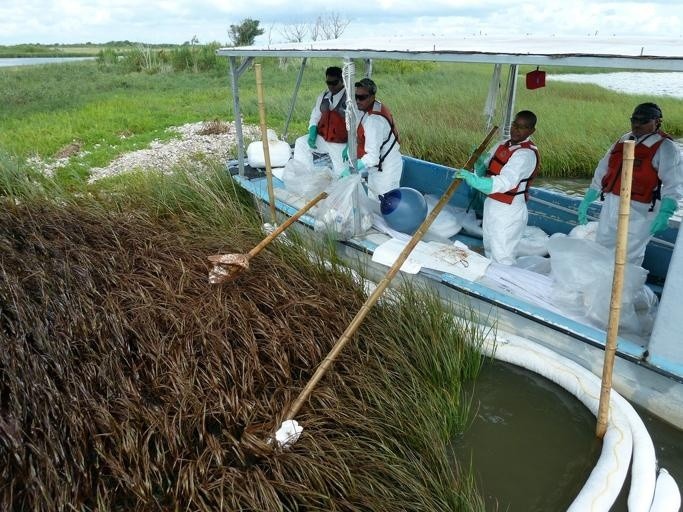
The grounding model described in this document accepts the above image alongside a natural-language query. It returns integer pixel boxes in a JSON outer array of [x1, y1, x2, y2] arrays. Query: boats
[[215, 36, 683, 430]]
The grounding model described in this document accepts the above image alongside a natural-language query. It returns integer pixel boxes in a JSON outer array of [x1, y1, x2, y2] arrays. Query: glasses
[[631, 117, 652, 125], [326, 79, 340, 86], [511, 121, 532, 131], [355, 94, 370, 101]]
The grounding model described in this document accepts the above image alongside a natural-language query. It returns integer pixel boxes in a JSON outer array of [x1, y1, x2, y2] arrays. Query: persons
[[339, 77, 405, 207], [576, 101, 683, 270], [450, 108, 541, 267], [290, 65, 356, 180]]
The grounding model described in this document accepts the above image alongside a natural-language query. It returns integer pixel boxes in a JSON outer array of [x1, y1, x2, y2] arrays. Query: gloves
[[649, 197, 678, 236], [342, 143, 349, 163], [308, 125, 318, 149], [341, 159, 366, 177], [472, 144, 487, 177], [452, 168, 493, 195], [577, 188, 601, 226]]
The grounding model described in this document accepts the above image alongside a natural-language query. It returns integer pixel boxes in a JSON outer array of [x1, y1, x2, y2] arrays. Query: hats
[[354, 78, 377, 95]]
